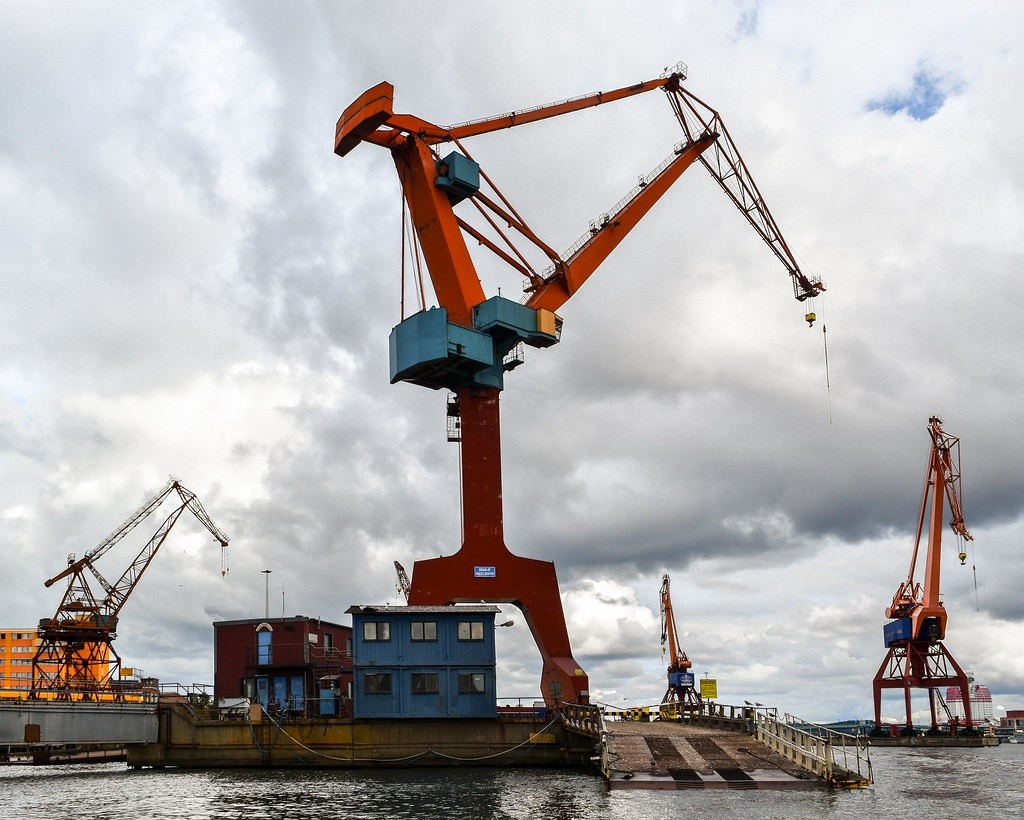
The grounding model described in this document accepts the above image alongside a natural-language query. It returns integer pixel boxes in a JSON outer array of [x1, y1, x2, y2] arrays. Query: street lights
[[261, 570, 273, 617], [704, 672, 710, 715]]
